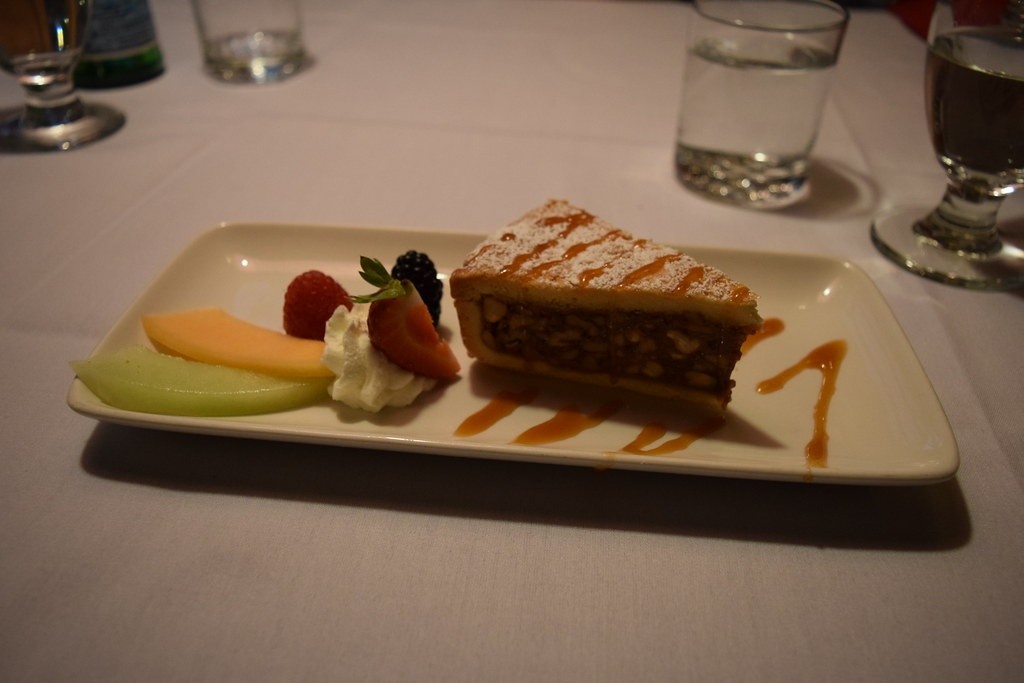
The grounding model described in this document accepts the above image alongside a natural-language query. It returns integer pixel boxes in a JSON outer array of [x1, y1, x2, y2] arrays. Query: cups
[[191, 1, 308, 84], [675, 1, 850, 208]]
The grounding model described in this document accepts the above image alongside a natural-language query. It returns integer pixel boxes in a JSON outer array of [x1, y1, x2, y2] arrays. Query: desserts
[[449, 199, 764, 413]]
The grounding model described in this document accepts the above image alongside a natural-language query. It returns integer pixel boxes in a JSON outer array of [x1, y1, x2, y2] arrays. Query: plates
[[69, 222, 958, 483]]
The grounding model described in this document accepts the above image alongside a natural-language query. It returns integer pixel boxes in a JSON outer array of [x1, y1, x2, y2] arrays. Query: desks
[[0, 0, 1024, 683]]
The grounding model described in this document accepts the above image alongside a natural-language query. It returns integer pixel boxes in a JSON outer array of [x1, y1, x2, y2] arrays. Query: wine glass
[[870, 1, 1024, 290], [0, 0, 125, 151]]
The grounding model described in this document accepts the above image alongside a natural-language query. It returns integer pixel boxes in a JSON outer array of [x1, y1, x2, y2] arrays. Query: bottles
[[68, 0, 162, 88]]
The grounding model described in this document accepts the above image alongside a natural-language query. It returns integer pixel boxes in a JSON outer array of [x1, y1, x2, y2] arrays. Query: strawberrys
[[356, 257, 463, 381]]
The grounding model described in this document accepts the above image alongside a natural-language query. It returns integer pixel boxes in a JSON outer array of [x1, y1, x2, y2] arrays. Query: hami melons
[[141, 308, 338, 378]]
